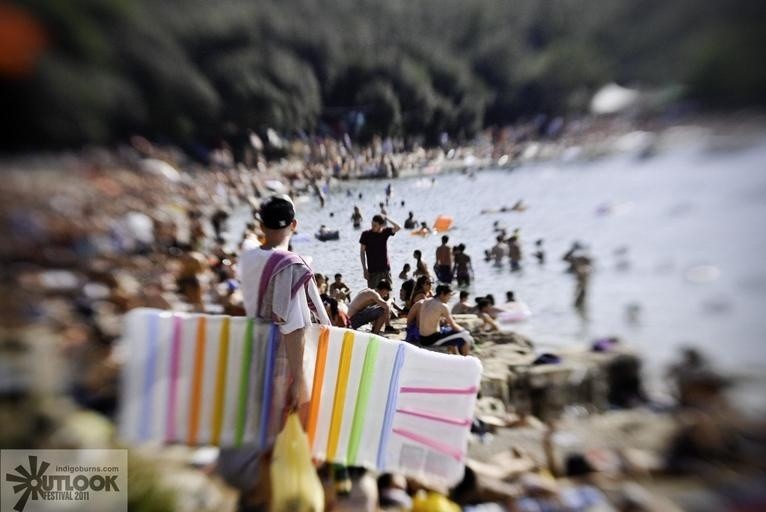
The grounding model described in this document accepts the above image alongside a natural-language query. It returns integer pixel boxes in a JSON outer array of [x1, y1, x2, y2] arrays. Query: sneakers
[[384, 325, 400, 334]]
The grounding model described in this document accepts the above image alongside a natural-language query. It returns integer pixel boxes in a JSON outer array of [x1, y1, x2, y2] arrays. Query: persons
[[1, 113, 766, 512]]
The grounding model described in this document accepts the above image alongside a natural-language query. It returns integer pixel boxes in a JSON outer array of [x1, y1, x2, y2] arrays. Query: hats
[[377, 279, 392, 291], [253, 194, 295, 228]]
